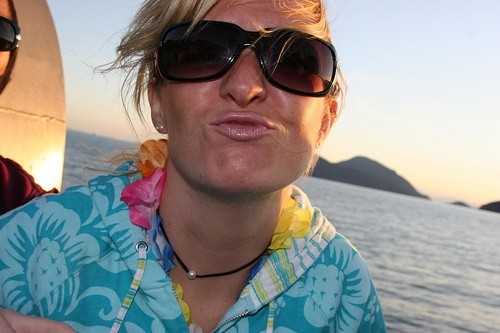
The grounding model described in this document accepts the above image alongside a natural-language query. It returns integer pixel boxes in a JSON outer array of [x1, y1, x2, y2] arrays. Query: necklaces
[[156, 208, 273, 279]]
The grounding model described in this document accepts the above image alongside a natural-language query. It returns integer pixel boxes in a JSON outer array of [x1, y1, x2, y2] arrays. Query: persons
[[0, 0, 61, 216], [0, 0, 386, 333]]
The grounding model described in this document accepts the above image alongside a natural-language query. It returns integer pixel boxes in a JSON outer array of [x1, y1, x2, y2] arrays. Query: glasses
[[0, 16, 21, 52], [154, 20, 341, 97]]
